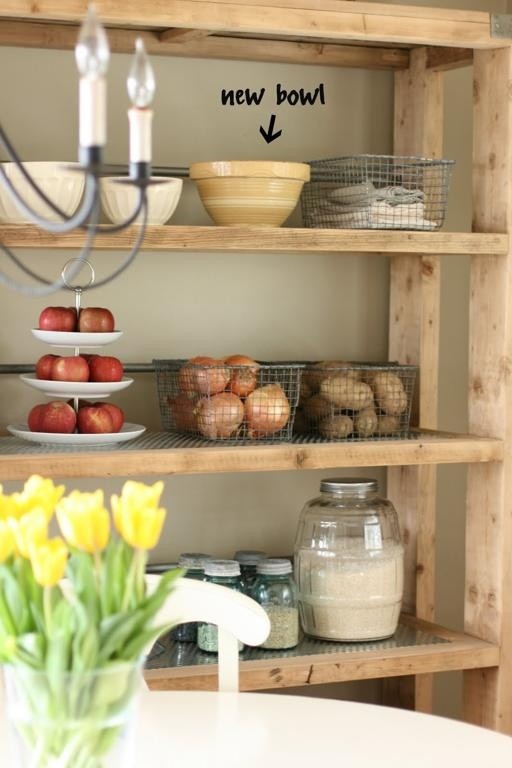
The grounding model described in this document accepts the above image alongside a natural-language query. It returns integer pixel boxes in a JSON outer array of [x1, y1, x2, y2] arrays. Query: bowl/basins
[[98, 176, 184, 225], [187, 161, 314, 226], [1, 160, 87, 226]]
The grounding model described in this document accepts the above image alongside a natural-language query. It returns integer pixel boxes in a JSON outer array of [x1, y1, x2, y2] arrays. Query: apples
[[28, 307, 123, 433]]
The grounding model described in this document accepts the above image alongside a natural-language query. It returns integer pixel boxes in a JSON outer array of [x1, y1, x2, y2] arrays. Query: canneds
[[179, 549, 302, 652]]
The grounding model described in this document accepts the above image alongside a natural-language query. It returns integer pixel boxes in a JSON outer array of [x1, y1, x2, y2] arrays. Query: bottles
[[249, 557, 300, 651], [292, 475, 405, 642], [231, 550, 266, 595], [167, 552, 213, 643], [201, 559, 247, 654]]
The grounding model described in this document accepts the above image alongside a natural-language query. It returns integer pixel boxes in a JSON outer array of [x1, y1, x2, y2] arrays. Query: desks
[[0, 690, 511, 767]]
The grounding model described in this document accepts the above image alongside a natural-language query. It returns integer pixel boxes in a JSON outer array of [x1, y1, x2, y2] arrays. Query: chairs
[[132, 571, 270, 691]]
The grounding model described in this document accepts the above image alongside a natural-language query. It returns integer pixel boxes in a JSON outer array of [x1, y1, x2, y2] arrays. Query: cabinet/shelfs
[[0, 0, 510, 736]]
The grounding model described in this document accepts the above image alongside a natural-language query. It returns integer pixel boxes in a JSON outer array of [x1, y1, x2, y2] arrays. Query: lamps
[[3, 0, 160, 292]]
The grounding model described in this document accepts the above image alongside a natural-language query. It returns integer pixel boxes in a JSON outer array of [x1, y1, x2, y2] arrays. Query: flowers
[[0, 474, 189, 757]]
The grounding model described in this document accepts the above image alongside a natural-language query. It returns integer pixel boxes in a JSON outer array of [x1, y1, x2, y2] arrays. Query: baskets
[[305, 362, 417, 440], [301, 153, 455, 232], [153, 357, 305, 443]]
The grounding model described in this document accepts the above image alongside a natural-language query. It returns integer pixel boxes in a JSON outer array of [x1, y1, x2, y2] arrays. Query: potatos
[[300, 362, 407, 436]]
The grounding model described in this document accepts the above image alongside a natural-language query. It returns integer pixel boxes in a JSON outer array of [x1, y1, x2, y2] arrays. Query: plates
[[20, 369, 136, 400], [30, 331, 126, 348], [5, 419, 148, 447]]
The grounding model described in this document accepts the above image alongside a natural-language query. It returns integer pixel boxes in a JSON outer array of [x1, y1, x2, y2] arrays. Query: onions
[[170, 353, 290, 438]]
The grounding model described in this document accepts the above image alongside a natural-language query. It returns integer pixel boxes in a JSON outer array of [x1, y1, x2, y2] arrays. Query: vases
[[0, 663, 139, 766]]
